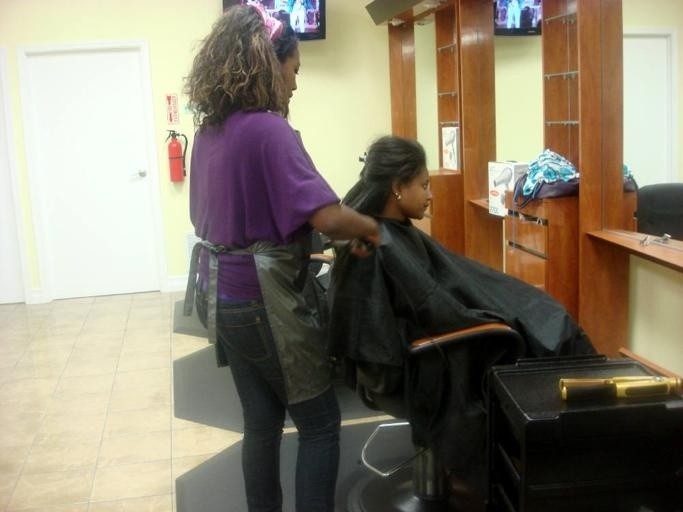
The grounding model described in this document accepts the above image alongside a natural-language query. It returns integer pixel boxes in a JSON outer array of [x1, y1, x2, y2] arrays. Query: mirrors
[[622, 0, 683, 241], [490, 0, 545, 166], [400, 7, 441, 173]]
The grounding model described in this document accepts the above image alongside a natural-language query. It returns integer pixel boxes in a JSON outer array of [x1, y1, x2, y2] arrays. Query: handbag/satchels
[[513, 147, 580, 209]]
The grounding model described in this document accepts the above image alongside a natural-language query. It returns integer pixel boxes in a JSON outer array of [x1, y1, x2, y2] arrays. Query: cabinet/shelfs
[[472, 350, 683, 512], [410, 169, 465, 259], [502, 190, 578, 325]]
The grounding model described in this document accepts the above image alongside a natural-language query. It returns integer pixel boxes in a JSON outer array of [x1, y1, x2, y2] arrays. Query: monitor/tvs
[[493, 0, 543, 36], [223, 0, 326, 40]]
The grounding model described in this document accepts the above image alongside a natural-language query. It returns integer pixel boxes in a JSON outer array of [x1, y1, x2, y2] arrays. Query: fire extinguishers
[[164, 129, 188, 182]]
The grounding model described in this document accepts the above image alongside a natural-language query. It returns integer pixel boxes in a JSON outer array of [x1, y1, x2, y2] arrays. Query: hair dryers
[[445, 131, 455, 154], [494, 167, 512, 193]]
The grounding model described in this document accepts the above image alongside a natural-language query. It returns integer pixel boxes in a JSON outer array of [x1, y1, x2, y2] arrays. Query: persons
[[325, 139, 592, 401], [184, 1, 383, 512]]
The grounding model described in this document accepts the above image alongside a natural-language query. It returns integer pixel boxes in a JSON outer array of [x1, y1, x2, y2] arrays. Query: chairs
[[343, 324, 521, 512], [635, 183, 683, 241]]
